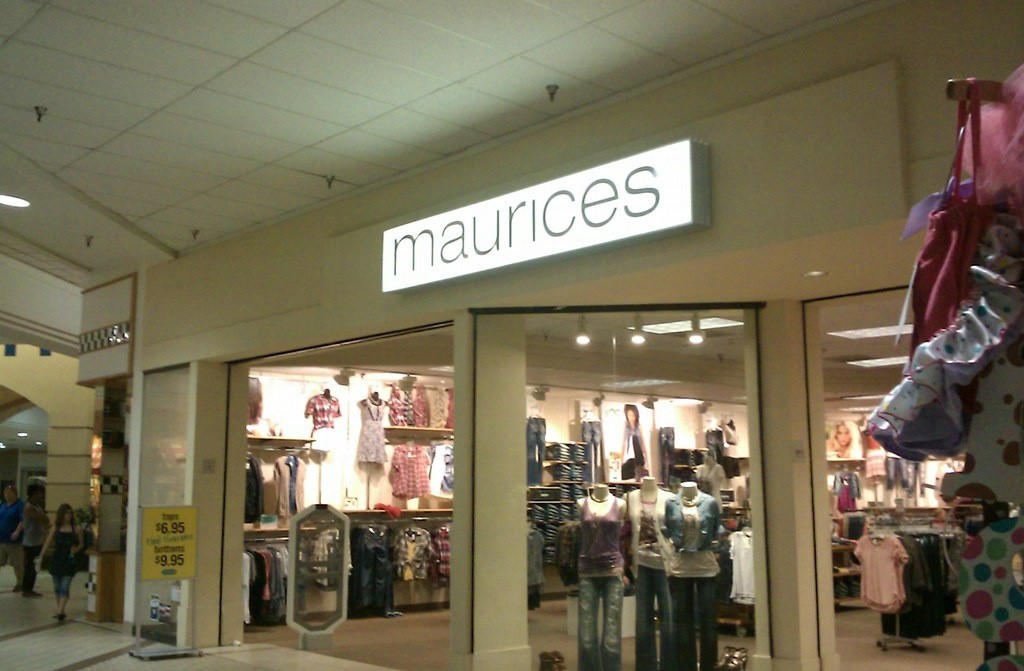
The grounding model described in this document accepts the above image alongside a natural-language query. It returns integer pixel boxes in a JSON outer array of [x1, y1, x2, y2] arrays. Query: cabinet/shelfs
[[832, 541, 863, 611], [525, 441, 590, 563]]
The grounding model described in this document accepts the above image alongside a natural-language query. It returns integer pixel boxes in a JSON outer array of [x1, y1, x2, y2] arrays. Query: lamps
[[687, 313, 706, 343], [642, 394, 658, 410], [630, 312, 647, 344], [593, 390, 605, 407], [531, 384, 549, 400], [697, 400, 713, 413], [574, 315, 592, 346], [334, 368, 355, 386], [398, 374, 417, 392]]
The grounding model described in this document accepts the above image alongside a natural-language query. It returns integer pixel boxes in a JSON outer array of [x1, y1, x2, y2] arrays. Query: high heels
[[717, 646, 737, 671], [723, 647, 748, 671]]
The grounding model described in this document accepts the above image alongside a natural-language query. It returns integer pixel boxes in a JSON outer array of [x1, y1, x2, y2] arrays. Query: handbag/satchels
[[67, 550, 89, 572]]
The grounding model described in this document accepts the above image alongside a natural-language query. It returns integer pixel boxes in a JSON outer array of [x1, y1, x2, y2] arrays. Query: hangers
[[242, 538, 286, 556], [246, 446, 253, 464], [361, 392, 388, 406], [866, 515, 964, 546], [405, 519, 421, 530], [739, 510, 752, 536]]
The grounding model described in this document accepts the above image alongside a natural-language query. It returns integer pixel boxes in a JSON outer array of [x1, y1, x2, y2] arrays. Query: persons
[[304, 389, 342, 451], [358, 393, 390, 464], [859, 412, 888, 477], [21, 483, 51, 597], [622, 476, 675, 671], [832, 421, 861, 458], [0, 484, 26, 592], [33, 503, 84, 623], [659, 481, 722, 671], [621, 404, 651, 481], [696, 455, 726, 514], [577, 483, 627, 671]]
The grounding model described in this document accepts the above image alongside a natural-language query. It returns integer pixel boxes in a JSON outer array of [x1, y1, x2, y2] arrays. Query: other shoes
[[22, 589, 42, 598], [538, 650, 568, 671], [52, 613, 67, 624], [12, 584, 23, 592]]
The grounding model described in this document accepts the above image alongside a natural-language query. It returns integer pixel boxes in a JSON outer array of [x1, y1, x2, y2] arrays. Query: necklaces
[[590, 493, 609, 503]]
